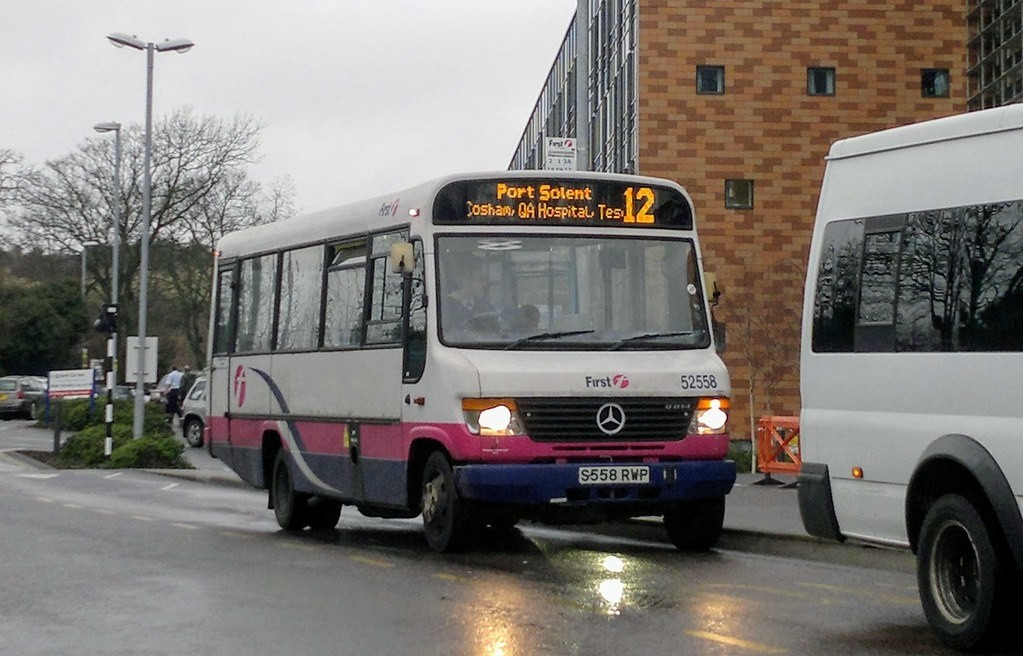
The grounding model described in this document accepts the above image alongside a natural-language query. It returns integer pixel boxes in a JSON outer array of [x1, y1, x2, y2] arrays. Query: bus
[[203, 170, 736, 553], [798, 106, 1023, 655]]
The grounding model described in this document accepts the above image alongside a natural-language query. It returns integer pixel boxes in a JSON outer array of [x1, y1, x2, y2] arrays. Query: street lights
[[81, 242, 99, 309], [93, 120, 121, 466], [105, 31, 195, 442]]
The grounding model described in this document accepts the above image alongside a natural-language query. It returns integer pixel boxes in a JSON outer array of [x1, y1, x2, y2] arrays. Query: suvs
[[178, 377, 207, 448], [0, 376, 48, 419]]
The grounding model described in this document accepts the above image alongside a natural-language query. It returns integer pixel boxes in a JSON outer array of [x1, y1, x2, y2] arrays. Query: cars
[[99, 386, 135, 401]]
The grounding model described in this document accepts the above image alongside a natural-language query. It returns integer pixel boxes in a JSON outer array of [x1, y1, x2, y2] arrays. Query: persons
[[441, 259, 539, 335], [143, 365, 197, 427]]
